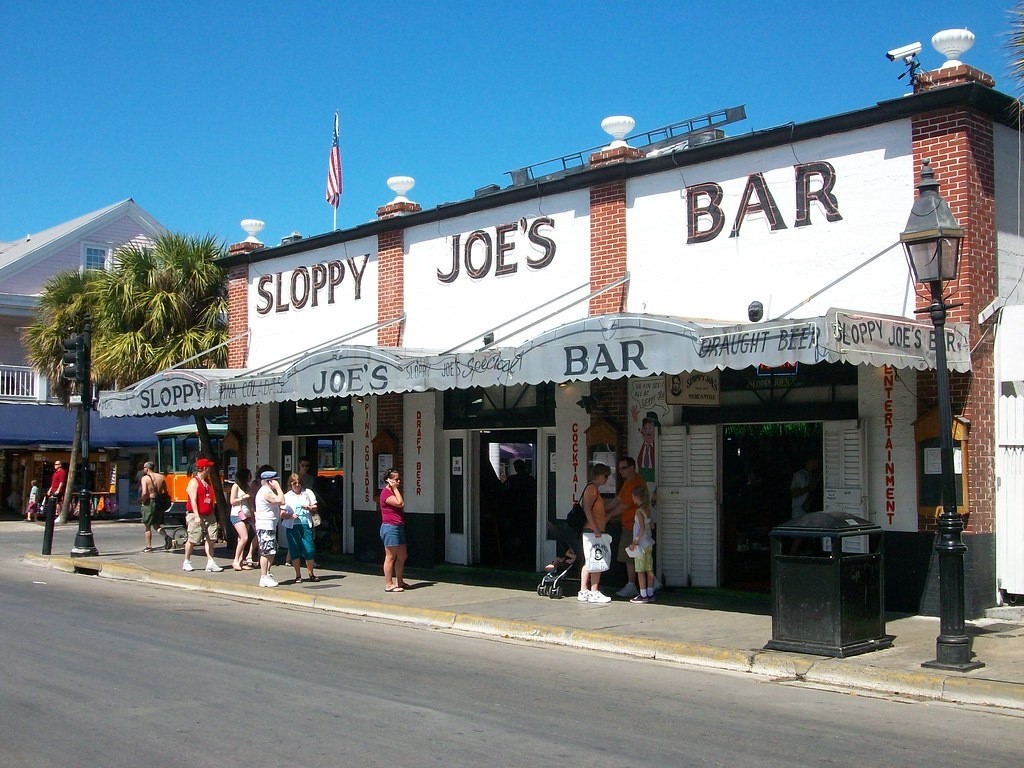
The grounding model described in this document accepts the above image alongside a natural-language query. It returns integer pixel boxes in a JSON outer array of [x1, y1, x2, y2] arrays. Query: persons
[[504, 459, 533, 495], [140, 462, 173, 553], [380, 468, 412, 591], [182, 458, 224, 573], [228, 456, 321, 587], [578, 456, 655, 603], [47, 460, 66, 516], [24, 480, 39, 521]]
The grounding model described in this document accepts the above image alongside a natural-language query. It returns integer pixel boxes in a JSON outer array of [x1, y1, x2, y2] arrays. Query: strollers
[[537, 534, 601, 600]]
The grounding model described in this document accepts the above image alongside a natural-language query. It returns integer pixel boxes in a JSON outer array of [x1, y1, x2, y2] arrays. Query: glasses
[[300, 463, 310, 468], [618, 464, 633, 471], [388, 469, 393, 477], [598, 474, 609, 479], [290, 482, 303, 485]]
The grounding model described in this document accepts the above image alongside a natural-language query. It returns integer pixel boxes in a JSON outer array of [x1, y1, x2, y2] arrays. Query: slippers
[[385, 586, 404, 592], [398, 583, 412, 589]]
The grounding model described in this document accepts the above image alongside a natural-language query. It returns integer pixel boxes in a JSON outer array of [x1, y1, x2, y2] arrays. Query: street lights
[[899, 157, 987, 674]]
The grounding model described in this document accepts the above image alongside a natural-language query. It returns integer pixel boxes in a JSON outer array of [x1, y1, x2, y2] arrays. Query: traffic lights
[[61, 335, 85, 382]]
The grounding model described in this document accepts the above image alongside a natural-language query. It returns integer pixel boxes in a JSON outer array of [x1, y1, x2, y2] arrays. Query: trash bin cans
[[766, 510, 895, 658]]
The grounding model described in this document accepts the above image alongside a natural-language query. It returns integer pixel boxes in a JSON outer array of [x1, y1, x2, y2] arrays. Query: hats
[[195, 458, 214, 468], [261, 470, 278, 480]]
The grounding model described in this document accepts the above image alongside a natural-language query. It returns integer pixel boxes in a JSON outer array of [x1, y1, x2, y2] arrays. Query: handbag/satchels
[[304, 487, 321, 527], [238, 510, 255, 521], [566, 483, 599, 530], [582, 532, 612, 572], [155, 493, 172, 511]]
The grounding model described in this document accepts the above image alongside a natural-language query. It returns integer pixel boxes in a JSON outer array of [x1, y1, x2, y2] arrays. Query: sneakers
[[616, 584, 639, 596], [259, 574, 278, 587], [629, 594, 648, 603], [578, 590, 591, 601], [646, 594, 656, 602], [588, 590, 611, 603], [183, 561, 194, 571], [205, 561, 224, 572]]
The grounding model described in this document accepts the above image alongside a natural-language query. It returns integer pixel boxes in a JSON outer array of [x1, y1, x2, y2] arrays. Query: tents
[[97, 239, 973, 422]]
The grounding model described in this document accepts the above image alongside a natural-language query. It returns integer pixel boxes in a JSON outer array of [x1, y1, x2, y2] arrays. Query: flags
[[326, 114, 342, 210]]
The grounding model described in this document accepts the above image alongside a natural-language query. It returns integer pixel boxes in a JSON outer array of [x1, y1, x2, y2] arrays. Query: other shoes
[[295, 576, 301, 583], [309, 576, 319, 582], [165, 536, 173, 550], [141, 546, 154, 554], [232, 555, 261, 571], [285, 561, 292, 566]]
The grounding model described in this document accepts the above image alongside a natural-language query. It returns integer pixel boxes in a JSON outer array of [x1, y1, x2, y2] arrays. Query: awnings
[[0, 405, 226, 447]]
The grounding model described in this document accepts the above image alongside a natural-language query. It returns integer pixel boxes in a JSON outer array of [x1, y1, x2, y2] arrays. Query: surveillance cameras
[[885, 41, 922, 62]]
